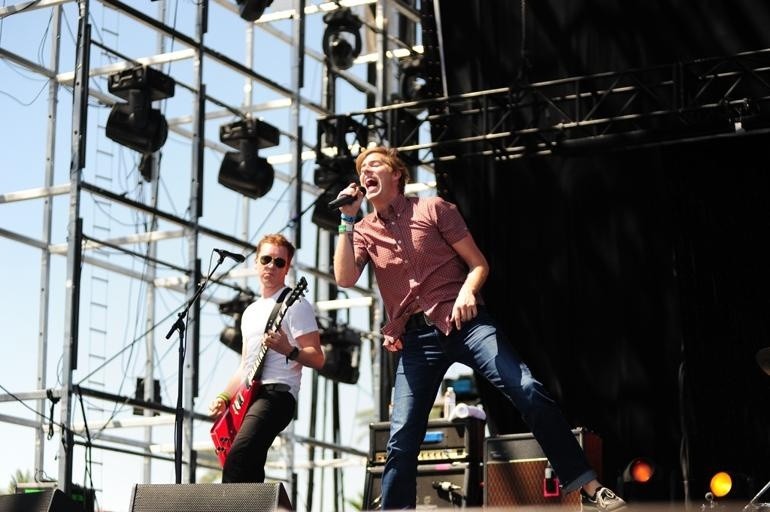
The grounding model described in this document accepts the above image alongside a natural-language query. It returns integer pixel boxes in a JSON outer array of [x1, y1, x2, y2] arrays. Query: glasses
[[259, 255, 287, 269]]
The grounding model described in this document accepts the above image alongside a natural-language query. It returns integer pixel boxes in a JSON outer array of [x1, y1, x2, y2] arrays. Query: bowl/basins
[[427, 404, 443, 419]]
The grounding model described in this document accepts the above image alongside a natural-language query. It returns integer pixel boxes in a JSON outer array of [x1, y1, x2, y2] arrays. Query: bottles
[[443, 385, 456, 422]]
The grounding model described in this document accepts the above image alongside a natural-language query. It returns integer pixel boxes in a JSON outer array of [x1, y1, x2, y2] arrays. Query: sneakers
[[578, 482, 626, 512]]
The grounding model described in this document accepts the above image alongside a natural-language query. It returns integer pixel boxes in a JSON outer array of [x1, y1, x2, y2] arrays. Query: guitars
[[210, 278, 308, 466]]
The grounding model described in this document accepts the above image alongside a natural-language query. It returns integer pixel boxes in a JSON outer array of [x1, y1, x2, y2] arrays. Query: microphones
[[214, 247, 244, 263], [329, 186, 367, 211], [433, 481, 461, 494]]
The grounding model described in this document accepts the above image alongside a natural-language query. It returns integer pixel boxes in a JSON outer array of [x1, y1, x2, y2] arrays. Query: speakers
[[127, 485, 292, 512], [0, 488, 74, 512], [361, 467, 469, 510], [482, 431, 586, 511]]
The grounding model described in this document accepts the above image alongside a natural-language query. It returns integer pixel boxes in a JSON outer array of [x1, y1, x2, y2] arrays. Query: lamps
[[396, 55, 445, 120], [217, 287, 255, 356], [234, 0, 276, 23], [315, 326, 363, 386], [103, 62, 177, 153], [320, 5, 366, 74], [310, 112, 371, 235], [622, 458, 657, 486], [216, 116, 281, 201]]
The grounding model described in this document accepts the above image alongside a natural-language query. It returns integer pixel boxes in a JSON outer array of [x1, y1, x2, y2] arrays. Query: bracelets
[[338, 224, 353, 234], [286, 347, 298, 365], [340, 213, 357, 222]]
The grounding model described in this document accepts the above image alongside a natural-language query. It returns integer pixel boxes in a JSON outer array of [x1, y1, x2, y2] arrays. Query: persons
[[206, 234, 324, 483], [331, 147, 629, 512]]
